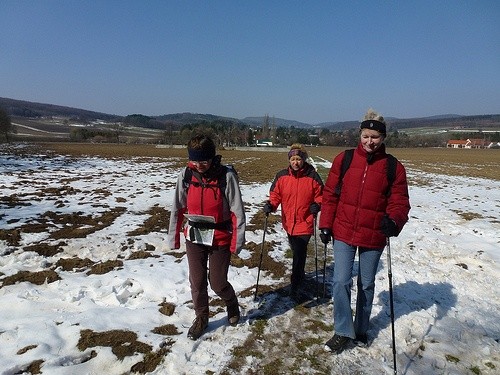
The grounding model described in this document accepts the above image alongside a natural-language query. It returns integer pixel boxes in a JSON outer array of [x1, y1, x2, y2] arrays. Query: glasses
[[190, 161, 209, 166]]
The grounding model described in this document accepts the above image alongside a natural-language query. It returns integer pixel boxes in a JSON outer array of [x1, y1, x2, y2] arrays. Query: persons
[[264, 143, 324, 286], [318, 111, 411, 353], [168, 132, 246, 341]]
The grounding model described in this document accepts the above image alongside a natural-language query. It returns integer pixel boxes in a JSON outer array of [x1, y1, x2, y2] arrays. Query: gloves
[[319, 230, 333, 245], [264, 203, 274, 213], [311, 203, 320, 214], [380, 216, 398, 236]]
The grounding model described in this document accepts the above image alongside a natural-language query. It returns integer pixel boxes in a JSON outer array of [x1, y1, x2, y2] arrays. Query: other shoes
[[354, 331, 368, 347], [324, 334, 349, 352], [187, 318, 209, 339], [227, 303, 240, 325]]
